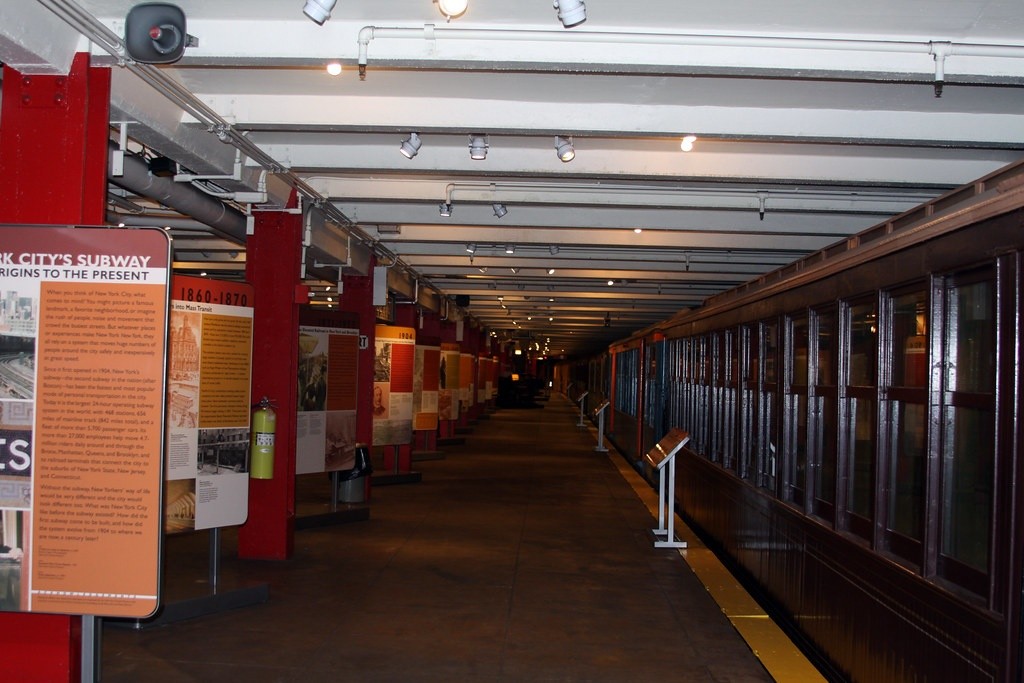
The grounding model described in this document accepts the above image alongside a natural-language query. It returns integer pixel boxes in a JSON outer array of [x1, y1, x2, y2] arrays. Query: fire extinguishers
[[250, 397, 277, 480]]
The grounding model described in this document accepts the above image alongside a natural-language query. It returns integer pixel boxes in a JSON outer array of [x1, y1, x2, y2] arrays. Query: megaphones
[[125, 3, 187, 65]]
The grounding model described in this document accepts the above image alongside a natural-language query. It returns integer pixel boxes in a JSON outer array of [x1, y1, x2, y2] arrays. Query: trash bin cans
[[336, 443, 367, 503]]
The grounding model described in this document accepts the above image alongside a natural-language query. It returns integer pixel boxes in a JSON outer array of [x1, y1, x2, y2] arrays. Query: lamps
[[505, 245, 516, 253], [303, 0, 337, 24], [554, 136, 575, 162], [479, 267, 557, 324], [439, 203, 454, 217], [549, 246, 560, 255], [433, 0, 468, 21], [467, 133, 489, 159], [201, 252, 212, 258], [493, 204, 508, 218], [229, 252, 239, 258], [399, 132, 422, 159], [467, 244, 477, 253], [553, 0, 587, 26]]
